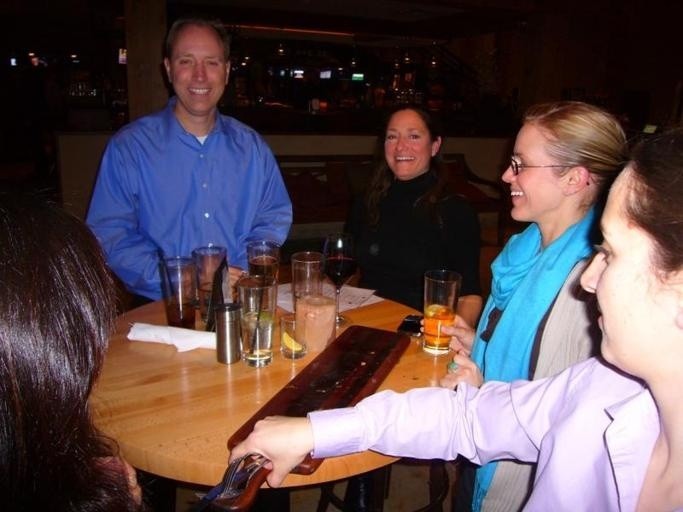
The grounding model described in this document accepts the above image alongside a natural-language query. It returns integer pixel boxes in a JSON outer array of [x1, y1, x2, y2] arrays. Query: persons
[[343, 109, 483, 334], [418, 98, 627, 512], [82, 12, 294, 301], [228, 124, 683, 512], [0, 192, 143, 511]]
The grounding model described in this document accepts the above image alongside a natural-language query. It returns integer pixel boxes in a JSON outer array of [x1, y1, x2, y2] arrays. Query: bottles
[[63, 48, 128, 132], [367, 54, 451, 123]]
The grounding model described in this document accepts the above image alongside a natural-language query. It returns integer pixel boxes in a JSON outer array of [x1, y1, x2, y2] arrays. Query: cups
[[423, 270, 463, 355], [158, 241, 323, 367]]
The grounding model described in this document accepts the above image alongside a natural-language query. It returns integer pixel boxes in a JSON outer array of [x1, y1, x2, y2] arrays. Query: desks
[[85, 282, 468, 512]]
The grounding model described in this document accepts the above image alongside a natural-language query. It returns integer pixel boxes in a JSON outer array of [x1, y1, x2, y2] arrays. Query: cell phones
[[397, 314, 423, 338]]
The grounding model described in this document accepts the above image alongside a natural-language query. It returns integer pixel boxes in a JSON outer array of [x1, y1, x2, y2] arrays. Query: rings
[[446, 360, 460, 371]]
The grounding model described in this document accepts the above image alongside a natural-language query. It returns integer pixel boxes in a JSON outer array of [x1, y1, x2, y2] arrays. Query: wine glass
[[323, 234, 357, 327]]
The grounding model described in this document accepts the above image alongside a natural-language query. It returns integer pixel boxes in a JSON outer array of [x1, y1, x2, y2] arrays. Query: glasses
[[510, 158, 590, 185]]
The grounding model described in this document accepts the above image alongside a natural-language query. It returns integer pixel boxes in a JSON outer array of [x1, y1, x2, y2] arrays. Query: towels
[[127, 322, 217, 353]]
